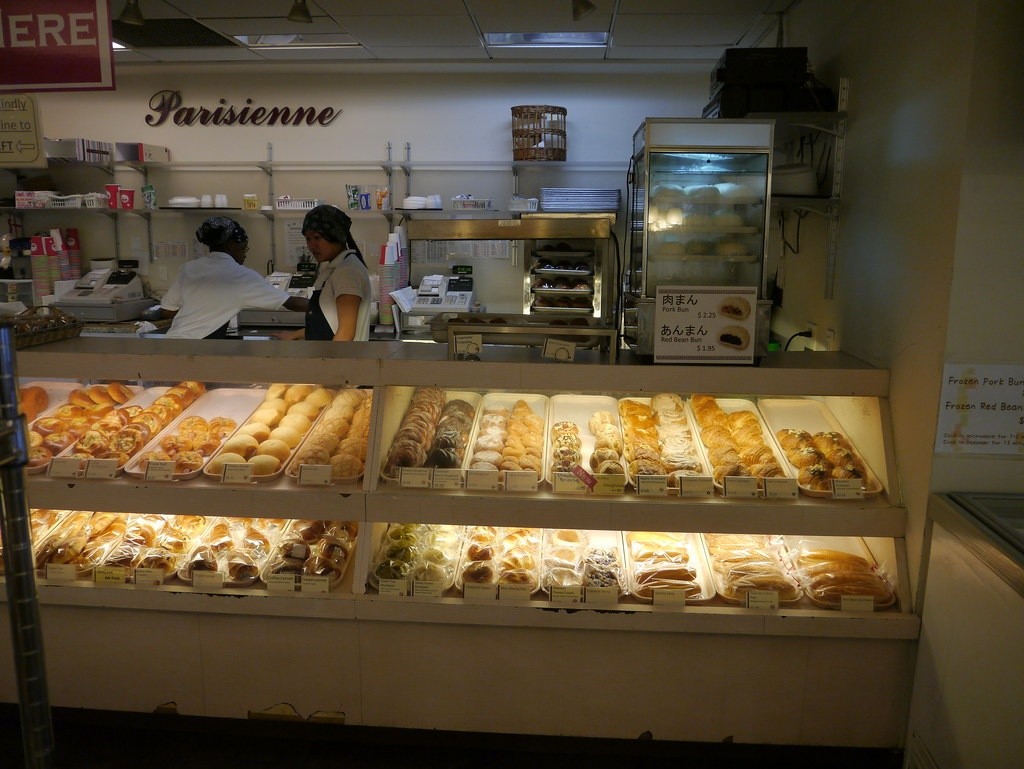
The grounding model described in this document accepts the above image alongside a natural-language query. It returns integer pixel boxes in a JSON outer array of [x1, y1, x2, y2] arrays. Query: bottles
[[360, 188, 371, 210]]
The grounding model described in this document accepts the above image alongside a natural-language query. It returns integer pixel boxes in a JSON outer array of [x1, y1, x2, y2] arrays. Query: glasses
[[242, 241, 249, 253]]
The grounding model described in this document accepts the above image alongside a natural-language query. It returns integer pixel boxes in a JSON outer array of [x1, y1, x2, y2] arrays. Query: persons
[[269, 205, 371, 341], [160, 217, 310, 339]]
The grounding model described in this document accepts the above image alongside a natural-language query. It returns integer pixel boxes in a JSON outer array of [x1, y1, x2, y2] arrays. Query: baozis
[[645, 181, 756, 258], [719, 297, 750, 320], [716, 326, 750, 349]]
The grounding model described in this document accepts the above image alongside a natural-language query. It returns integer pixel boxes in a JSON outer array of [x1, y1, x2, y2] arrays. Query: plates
[[533, 268, 590, 275], [402, 196, 426, 209], [532, 306, 592, 312], [532, 288, 591, 294], [541, 187, 621, 212], [168, 195, 200, 208], [536, 250, 592, 257]]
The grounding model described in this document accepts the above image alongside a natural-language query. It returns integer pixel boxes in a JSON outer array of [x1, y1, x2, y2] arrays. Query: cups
[[104, 184, 121, 208], [379, 225, 410, 325], [201, 193, 228, 207], [119, 189, 135, 208], [427, 194, 441, 208], [31, 228, 81, 307]]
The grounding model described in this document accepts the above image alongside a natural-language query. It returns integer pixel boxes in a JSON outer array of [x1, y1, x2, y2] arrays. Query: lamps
[[570, 0, 597, 23], [118, 0, 145, 25], [286, 0, 313, 26]]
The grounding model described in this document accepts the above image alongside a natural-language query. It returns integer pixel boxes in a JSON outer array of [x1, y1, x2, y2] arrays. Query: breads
[[18, 378, 372, 585], [382, 383, 869, 493], [370, 524, 894, 606]]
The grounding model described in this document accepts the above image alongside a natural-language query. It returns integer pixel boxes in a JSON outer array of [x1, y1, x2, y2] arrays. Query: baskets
[[48, 194, 83, 209], [14, 320, 86, 348], [86, 194, 107, 208]]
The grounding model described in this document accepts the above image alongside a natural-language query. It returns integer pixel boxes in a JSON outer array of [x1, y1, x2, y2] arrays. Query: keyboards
[[64, 289, 114, 299], [415, 295, 467, 307]]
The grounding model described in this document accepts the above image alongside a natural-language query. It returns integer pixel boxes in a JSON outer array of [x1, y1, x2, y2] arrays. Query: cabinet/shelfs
[[13, 335, 917, 638], [631, 119, 776, 358]]
